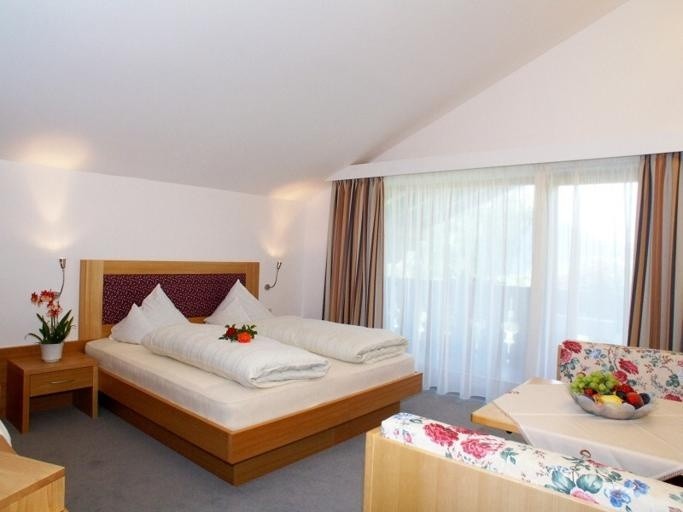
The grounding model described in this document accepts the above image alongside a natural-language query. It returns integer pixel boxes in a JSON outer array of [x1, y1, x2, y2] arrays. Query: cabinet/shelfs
[[0, 454, 65, 512]]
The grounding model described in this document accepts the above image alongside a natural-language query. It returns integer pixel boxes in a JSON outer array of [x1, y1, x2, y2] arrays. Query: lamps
[[57, 259, 66, 297], [265, 263, 281, 289]]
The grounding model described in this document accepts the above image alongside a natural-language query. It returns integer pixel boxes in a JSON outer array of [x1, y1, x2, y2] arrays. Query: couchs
[[557, 340, 683, 401], [362, 412, 683, 512]]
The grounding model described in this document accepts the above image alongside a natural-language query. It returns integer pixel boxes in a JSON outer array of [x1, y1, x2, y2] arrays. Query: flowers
[[25, 290, 74, 344]]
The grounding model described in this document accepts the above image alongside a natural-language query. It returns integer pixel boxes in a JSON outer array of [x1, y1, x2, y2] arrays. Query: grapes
[[570, 372, 617, 394]]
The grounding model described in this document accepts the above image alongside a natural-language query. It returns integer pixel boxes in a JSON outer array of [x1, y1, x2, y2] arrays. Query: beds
[[81, 259, 421, 485]]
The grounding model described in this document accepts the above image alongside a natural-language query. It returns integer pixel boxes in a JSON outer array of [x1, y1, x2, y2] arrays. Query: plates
[[568, 383, 656, 420]]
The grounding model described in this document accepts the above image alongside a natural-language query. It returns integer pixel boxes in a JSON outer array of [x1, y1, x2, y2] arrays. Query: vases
[[40, 342, 63, 363]]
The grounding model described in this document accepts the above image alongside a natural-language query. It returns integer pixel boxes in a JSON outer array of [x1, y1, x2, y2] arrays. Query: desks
[[471, 378, 683, 481], [5, 351, 98, 433]]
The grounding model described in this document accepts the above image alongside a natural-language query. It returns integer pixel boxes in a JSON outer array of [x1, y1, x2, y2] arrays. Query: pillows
[[110, 278, 274, 345]]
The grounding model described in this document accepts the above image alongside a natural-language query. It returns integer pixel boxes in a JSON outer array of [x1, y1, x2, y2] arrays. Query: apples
[[227, 328, 236, 337], [584, 384, 644, 409]]
